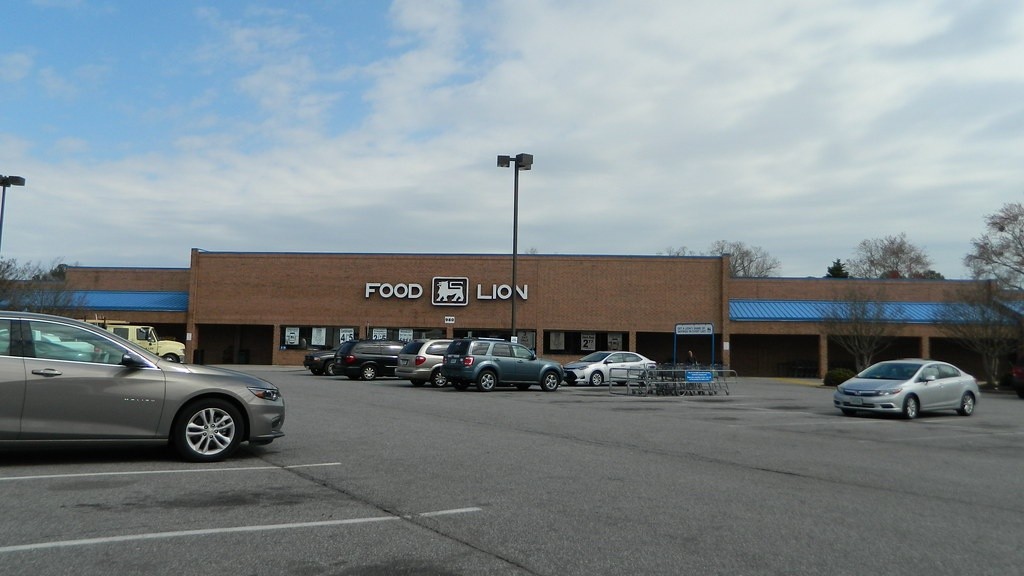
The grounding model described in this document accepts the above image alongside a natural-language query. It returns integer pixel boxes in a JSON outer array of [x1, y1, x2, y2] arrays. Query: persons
[[685, 350, 699, 366]]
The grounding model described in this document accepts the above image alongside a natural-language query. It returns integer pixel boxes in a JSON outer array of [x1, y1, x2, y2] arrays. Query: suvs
[[103, 324, 185, 364], [395, 339, 455, 388], [442, 337, 564, 391], [334, 339, 408, 381]]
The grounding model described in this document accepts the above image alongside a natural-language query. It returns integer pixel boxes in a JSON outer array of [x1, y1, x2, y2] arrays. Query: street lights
[[0, 175, 26, 255]]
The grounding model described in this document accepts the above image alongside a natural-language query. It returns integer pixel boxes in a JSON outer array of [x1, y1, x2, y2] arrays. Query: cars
[[562, 350, 657, 387], [303, 341, 347, 376], [832, 359, 981, 420], [0, 309, 285, 462]]
